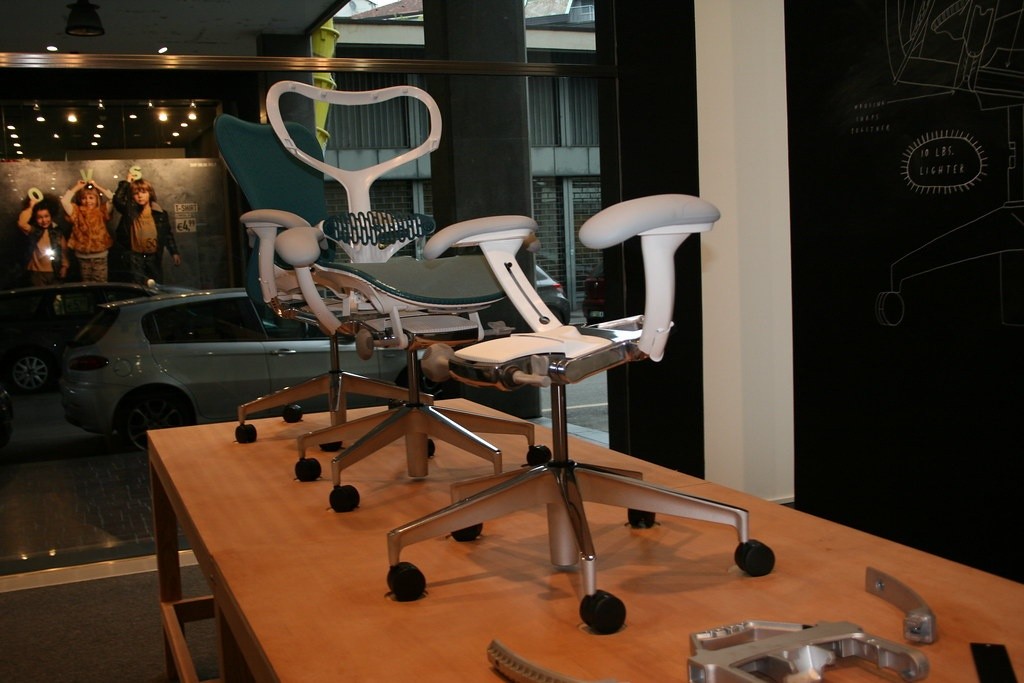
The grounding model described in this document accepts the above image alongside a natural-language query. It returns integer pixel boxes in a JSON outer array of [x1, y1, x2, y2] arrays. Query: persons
[[112, 173, 182, 285], [17, 199, 70, 286], [59, 179, 115, 283]]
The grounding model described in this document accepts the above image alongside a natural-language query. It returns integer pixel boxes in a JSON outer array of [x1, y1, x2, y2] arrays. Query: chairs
[[214, 115, 554, 515]]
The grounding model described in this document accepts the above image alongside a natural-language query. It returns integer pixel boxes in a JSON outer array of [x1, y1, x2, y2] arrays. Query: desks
[[146, 394, 1024, 683]]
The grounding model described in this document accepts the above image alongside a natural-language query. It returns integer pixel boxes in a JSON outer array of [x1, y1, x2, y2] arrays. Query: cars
[[534, 266, 572, 327], [0, 280, 203, 393], [58, 288, 481, 455], [582, 262, 607, 326]]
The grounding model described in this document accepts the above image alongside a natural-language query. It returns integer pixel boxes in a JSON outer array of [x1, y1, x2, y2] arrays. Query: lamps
[[66, 0, 104, 36]]
